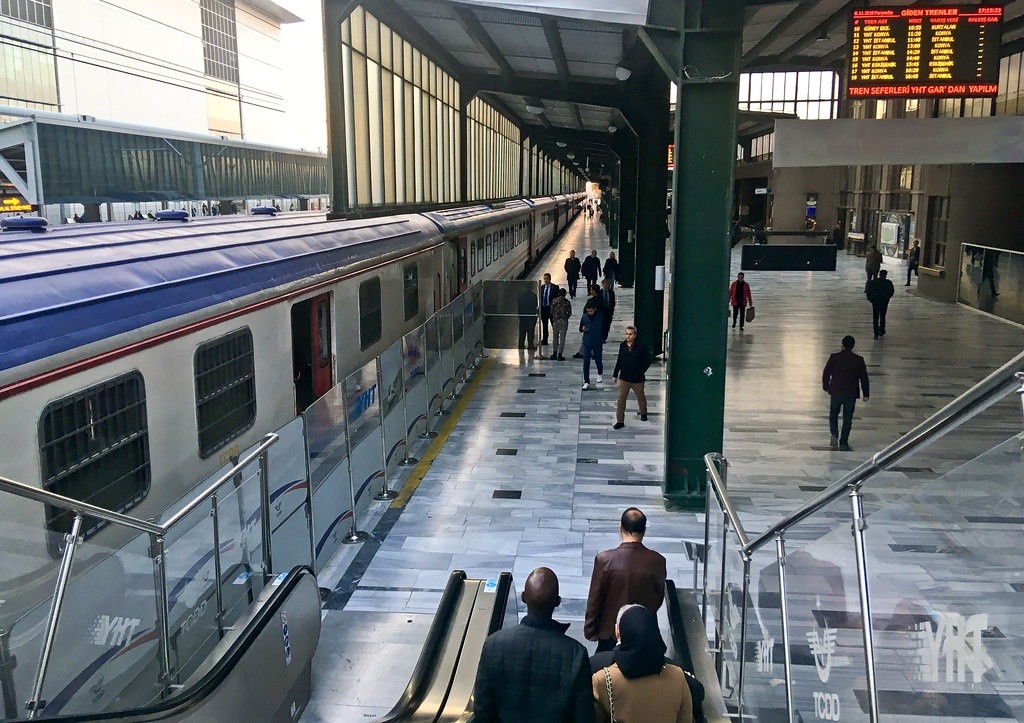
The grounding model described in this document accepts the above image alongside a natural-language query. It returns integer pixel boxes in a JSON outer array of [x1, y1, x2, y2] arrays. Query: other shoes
[[613, 422, 624, 429], [540, 340, 549, 345], [732, 322, 736, 328], [581, 382, 590, 390], [641, 414, 647, 421], [874, 335, 879, 340], [518, 344, 527, 349], [529, 345, 538, 349], [597, 374, 602, 383], [572, 352, 583, 358], [839, 445, 853, 452], [740, 326, 745, 331], [830, 437, 839, 445]]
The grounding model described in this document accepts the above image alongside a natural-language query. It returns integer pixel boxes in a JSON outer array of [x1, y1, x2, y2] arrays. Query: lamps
[[525, 97, 544, 115], [615, 59, 631, 80], [568, 153, 605, 182], [557, 140, 568, 148], [818, 30, 829, 43], [608, 120, 618, 132]]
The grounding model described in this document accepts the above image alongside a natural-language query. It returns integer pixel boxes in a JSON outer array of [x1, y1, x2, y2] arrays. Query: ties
[[604, 290, 607, 306], [544, 285, 549, 307]]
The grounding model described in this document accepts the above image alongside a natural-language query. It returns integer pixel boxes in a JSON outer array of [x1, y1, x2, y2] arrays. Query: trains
[[0, 192, 588, 723]]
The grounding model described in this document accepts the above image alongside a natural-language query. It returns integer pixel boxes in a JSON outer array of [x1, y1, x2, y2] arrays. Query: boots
[[550, 351, 557, 359], [558, 353, 565, 361]]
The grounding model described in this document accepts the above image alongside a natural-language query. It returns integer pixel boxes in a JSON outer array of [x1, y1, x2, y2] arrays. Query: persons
[[729, 272, 753, 331], [474, 567, 592, 723], [564, 250, 582, 298], [905, 240, 920, 286], [613, 326, 651, 429], [714, 542, 847, 723], [865, 246, 883, 292], [833, 225, 842, 249], [583, 250, 602, 295], [592, 606, 693, 723], [584, 204, 594, 218], [518, 282, 537, 349], [591, 604, 705, 723], [538, 273, 559, 346], [752, 224, 767, 244], [823, 335, 870, 449], [572, 279, 615, 389], [867, 270, 894, 340], [65, 201, 294, 223], [603, 252, 617, 290], [584, 507, 667, 653], [550, 288, 571, 361], [977, 249, 1000, 298]]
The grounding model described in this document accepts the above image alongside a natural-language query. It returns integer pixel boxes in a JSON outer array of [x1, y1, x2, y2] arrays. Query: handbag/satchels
[[746, 306, 755, 322]]
